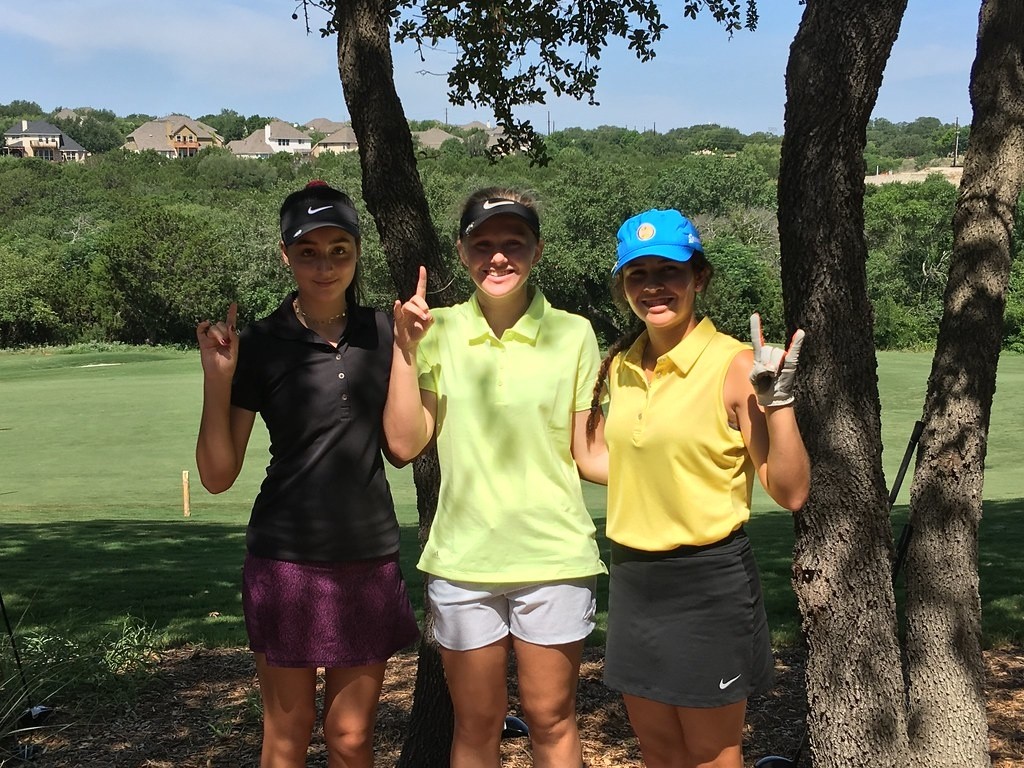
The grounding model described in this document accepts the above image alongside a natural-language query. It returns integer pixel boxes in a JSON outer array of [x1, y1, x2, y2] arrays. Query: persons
[[198, 180, 437, 768], [384, 188, 608, 768], [585, 209, 809, 768]]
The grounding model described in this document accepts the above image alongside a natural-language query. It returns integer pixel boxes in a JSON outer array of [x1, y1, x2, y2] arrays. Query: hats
[[611, 208, 704, 278], [280, 180, 360, 246], [459, 198, 539, 243]]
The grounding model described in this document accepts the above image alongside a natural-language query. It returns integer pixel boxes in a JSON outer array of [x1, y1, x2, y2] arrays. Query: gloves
[[749, 312, 805, 407]]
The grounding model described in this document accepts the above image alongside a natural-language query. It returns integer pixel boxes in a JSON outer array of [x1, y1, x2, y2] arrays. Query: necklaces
[[294, 298, 348, 325]]
